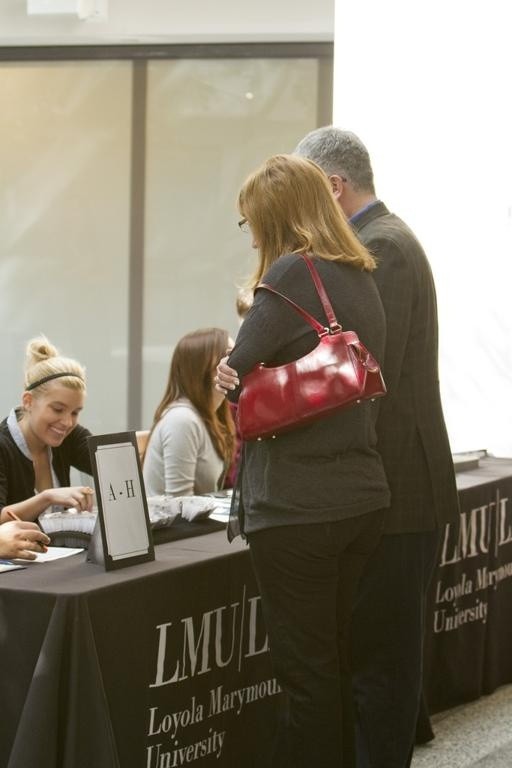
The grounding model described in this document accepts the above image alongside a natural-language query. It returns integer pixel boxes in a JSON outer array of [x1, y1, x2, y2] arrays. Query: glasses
[[239, 217, 248, 233]]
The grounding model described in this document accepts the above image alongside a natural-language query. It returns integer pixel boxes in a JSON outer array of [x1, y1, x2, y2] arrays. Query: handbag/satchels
[[236, 331, 387, 442]]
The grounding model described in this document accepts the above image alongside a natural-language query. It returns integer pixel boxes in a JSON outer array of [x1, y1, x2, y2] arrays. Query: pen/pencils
[[7, 511, 44, 546]]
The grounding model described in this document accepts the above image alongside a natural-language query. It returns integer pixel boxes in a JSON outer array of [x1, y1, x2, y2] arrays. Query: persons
[[210, 153, 390, 768], [0, 338, 96, 526], [0, 520, 52, 560], [291, 126, 460, 767], [141, 328, 236, 500]]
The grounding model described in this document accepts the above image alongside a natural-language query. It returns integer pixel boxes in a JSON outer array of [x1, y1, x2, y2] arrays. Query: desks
[[0, 450, 512, 768]]
